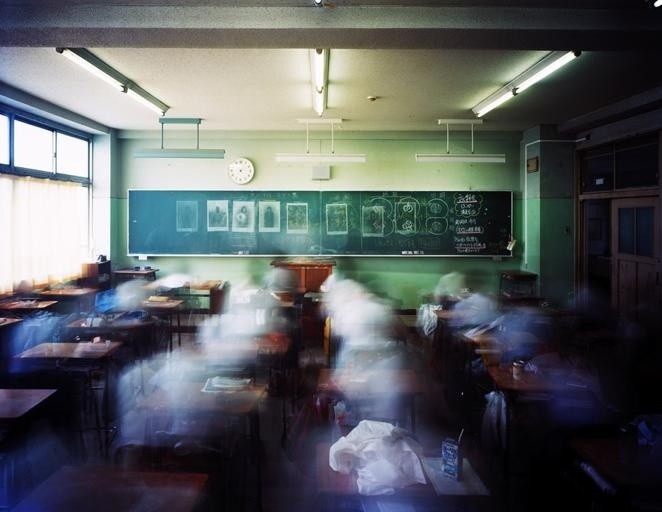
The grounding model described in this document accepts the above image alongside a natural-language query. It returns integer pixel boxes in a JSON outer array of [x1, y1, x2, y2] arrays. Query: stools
[[0, 257, 662, 512]]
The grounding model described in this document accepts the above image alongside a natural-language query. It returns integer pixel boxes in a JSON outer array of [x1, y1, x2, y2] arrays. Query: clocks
[[228, 157, 255, 186]]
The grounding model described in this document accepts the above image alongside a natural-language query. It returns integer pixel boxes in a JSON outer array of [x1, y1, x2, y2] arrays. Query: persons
[[10, 259, 608, 511]]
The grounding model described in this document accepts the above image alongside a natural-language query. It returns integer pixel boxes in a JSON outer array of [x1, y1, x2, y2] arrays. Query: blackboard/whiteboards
[[126, 190, 514, 258]]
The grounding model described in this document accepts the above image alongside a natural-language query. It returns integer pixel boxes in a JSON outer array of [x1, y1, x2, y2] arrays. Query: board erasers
[[238, 251, 250, 255], [401, 251, 424, 254]]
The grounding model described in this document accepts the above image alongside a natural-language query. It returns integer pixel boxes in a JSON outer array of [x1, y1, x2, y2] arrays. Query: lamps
[[56, 48, 171, 117], [412, 116, 508, 162], [272, 117, 369, 162], [131, 114, 226, 162], [308, 48, 331, 117], [468, 47, 581, 116]]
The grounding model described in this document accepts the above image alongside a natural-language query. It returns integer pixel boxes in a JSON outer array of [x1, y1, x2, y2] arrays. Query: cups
[[512, 360, 525, 380]]
